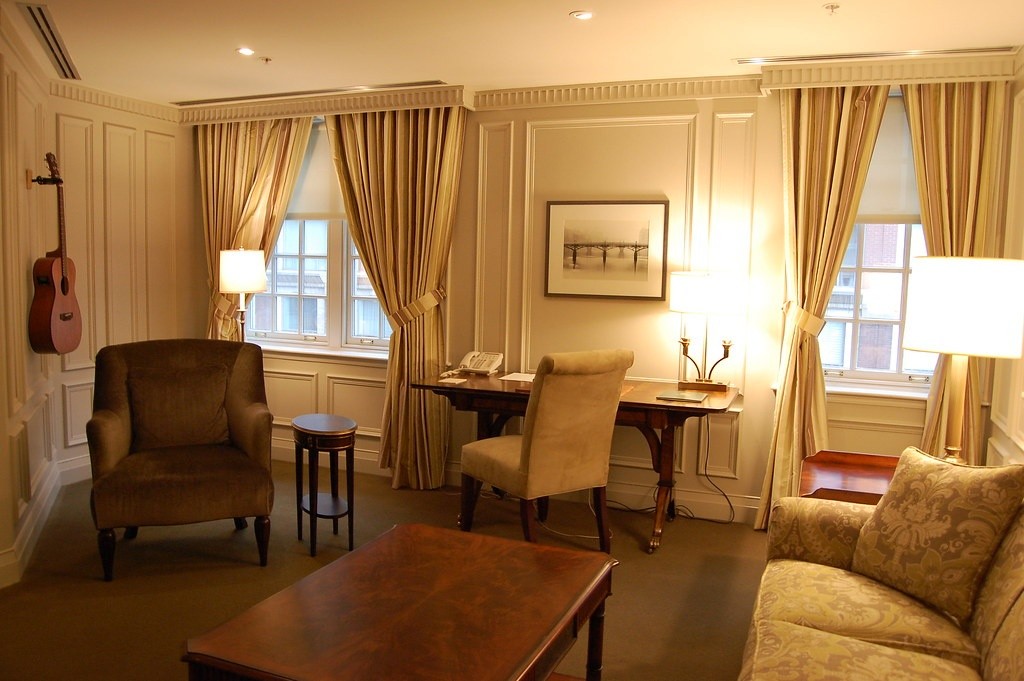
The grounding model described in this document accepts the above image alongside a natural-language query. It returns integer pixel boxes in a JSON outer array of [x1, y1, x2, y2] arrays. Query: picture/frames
[[544, 200, 669, 302]]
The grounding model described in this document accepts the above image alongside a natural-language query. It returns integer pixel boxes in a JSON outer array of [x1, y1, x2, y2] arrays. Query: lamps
[[219, 245, 268, 342], [902, 256, 1024, 466], [669, 272, 752, 393]]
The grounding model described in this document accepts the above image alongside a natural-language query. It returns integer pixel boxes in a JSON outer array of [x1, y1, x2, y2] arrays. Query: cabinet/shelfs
[[291, 413, 358, 558]]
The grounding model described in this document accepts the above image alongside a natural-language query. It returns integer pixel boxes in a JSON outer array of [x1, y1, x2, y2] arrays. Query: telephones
[[458, 351, 504, 376]]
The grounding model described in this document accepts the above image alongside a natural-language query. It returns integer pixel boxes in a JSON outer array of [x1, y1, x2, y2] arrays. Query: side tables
[[796, 449, 900, 506]]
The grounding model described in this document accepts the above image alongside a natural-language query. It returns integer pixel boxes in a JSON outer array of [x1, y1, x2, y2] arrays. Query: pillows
[[851, 445, 1024, 623]]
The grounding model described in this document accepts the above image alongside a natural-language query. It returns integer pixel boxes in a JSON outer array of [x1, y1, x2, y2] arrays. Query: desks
[[408, 371, 740, 554]]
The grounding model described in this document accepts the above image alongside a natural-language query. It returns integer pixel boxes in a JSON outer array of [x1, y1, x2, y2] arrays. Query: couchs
[[85, 337, 275, 581], [733, 497, 1024, 681]]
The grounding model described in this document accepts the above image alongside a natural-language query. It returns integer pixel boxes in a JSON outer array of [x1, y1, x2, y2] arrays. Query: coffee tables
[[181, 522, 621, 681]]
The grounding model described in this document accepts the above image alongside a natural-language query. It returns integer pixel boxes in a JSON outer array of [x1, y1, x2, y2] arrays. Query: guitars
[[26, 151, 83, 354]]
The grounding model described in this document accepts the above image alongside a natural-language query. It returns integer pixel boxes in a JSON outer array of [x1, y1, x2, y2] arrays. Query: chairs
[[460, 346, 635, 554]]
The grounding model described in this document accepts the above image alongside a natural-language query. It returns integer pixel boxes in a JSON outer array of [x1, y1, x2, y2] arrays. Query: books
[[656, 390, 708, 403]]
